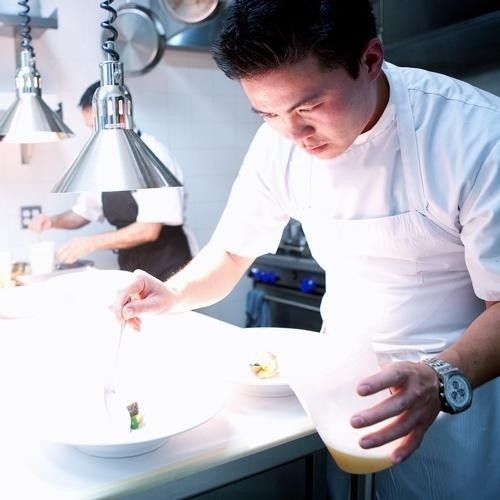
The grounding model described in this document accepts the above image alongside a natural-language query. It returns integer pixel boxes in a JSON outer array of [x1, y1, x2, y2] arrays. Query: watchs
[[422, 356, 475, 415]]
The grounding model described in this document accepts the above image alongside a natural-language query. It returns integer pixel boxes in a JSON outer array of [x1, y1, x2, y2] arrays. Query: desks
[[0, 269, 325, 500]]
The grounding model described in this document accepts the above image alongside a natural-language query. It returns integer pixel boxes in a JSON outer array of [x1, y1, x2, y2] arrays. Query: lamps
[[52, 0, 183, 197], [0, 0, 74, 144]]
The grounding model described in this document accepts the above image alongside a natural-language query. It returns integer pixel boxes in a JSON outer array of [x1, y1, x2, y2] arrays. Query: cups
[[283, 328, 410, 477], [24, 239, 54, 277]]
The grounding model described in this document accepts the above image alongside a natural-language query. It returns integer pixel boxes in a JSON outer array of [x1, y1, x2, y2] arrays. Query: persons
[[27, 79, 195, 280], [108, 0, 498, 500]]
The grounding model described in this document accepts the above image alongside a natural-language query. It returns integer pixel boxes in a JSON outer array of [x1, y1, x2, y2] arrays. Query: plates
[[214, 327, 328, 403], [37, 380, 227, 459]]
